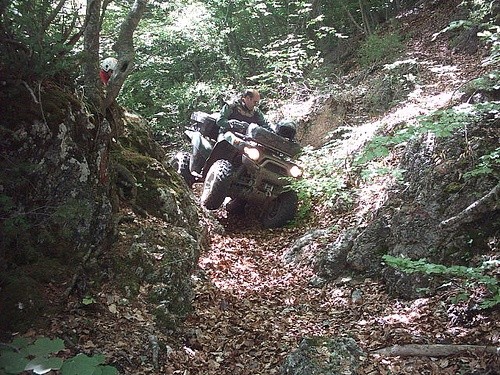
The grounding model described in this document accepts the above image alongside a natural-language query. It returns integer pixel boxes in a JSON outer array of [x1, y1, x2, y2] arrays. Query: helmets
[[101, 57, 119, 72], [276, 119, 297, 139]]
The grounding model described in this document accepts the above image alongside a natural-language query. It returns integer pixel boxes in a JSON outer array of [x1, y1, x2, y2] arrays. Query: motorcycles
[[172, 110, 302, 227]]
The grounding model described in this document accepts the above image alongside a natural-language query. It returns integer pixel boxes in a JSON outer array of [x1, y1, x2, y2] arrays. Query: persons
[[216, 88, 275, 154], [98, 56, 118, 85]]
